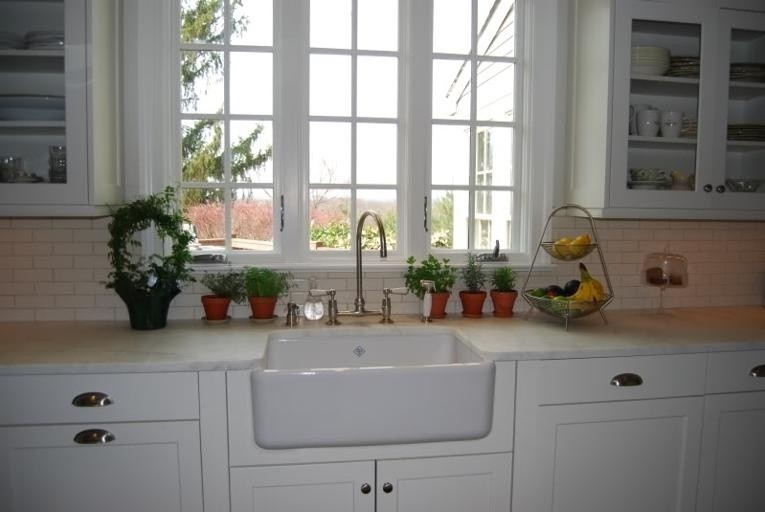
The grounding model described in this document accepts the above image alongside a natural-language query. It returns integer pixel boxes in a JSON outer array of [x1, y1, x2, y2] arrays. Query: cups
[[671, 170, 696, 190], [629, 168, 670, 180], [628, 103, 691, 137], [0, 154, 25, 181], [47, 142, 67, 183]]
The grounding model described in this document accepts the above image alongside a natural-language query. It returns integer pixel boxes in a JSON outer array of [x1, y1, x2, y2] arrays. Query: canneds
[[50, 146, 66, 182]]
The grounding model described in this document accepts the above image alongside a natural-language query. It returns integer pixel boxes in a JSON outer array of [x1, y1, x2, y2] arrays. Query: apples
[[552, 296, 568, 315], [532, 289, 543, 297], [539, 297, 552, 311], [567, 309, 581, 319]]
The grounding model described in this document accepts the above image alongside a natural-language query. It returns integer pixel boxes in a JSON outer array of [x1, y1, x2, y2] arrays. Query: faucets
[[352, 210, 388, 314]]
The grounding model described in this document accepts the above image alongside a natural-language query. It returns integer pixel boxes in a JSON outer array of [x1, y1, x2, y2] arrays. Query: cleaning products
[[286, 291, 300, 326]]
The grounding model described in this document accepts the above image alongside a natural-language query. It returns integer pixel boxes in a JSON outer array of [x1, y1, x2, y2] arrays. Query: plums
[[565, 281, 579, 297], [546, 284, 563, 295]]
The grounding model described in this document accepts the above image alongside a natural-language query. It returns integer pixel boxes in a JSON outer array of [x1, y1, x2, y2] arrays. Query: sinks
[[249, 325, 493, 450]]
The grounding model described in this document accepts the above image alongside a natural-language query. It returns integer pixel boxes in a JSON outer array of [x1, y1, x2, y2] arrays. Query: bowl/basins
[[725, 177, 761, 192]]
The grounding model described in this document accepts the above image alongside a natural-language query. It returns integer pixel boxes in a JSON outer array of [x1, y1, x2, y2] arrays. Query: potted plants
[[241, 266, 299, 318], [97, 184, 196, 330], [197, 267, 246, 319], [404, 255, 456, 319], [488, 265, 518, 314], [459, 251, 489, 313]]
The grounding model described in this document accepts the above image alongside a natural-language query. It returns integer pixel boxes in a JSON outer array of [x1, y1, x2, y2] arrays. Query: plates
[[630, 44, 671, 75], [681, 119, 698, 138], [729, 62, 765, 83], [0, 93, 66, 122], [628, 180, 663, 190], [24, 29, 66, 50], [668, 54, 700, 78], [0, 30, 23, 49], [726, 125, 765, 141]]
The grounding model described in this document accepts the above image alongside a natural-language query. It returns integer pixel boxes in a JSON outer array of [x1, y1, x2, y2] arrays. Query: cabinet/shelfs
[[512, 351, 765, 509], [0, 1, 121, 219], [229, 451, 510, 510], [572, 1, 765, 224], [1, 370, 205, 510]]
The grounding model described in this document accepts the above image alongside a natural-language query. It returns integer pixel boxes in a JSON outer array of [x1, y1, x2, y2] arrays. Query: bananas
[[568, 263, 604, 311]]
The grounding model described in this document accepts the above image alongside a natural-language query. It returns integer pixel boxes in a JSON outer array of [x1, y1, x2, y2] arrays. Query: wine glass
[[637, 252, 690, 316]]
